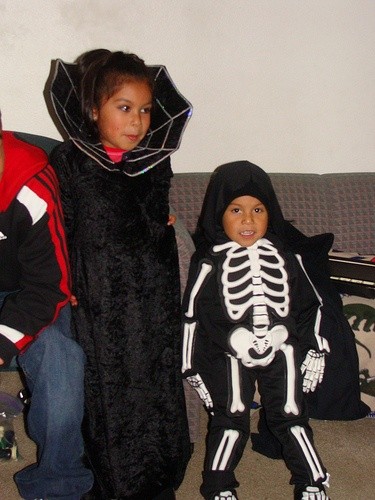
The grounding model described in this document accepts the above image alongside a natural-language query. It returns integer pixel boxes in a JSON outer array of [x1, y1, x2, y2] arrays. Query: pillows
[[328, 248, 375, 416]]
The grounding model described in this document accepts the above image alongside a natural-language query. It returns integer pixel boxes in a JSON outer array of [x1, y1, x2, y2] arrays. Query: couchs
[[168, 171, 375, 306]]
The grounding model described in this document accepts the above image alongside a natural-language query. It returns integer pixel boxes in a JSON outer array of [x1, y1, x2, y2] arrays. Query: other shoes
[[290, 478, 330, 500], [200, 471, 240, 500]]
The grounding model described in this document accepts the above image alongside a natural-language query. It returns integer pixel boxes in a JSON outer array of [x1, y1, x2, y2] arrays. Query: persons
[[179, 167, 333, 500], [0, 112, 96, 500], [47, 52, 187, 500]]
